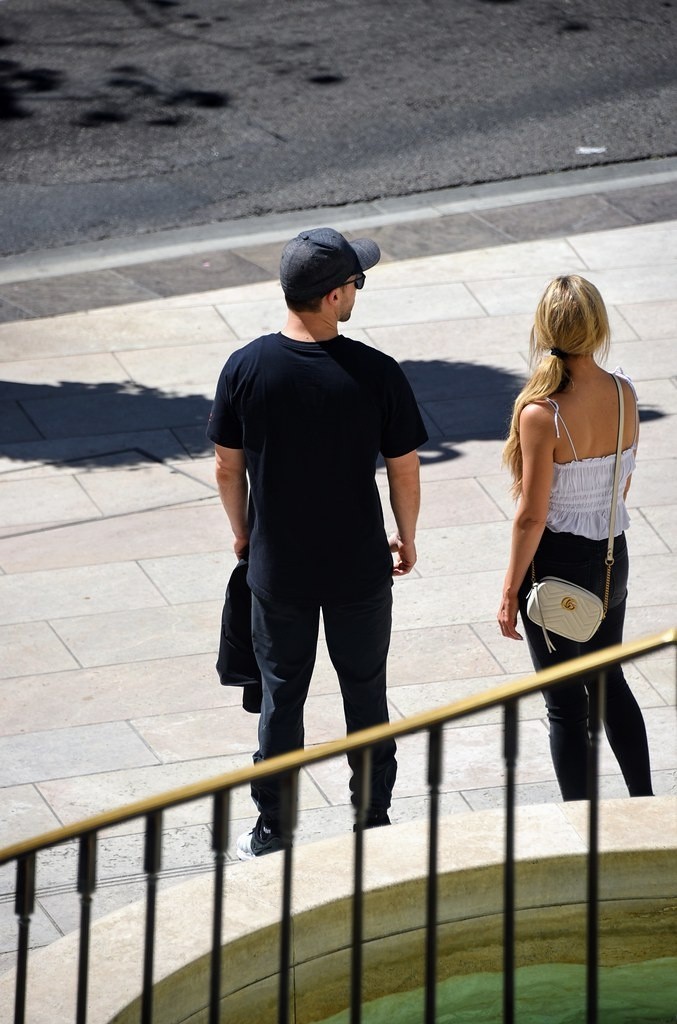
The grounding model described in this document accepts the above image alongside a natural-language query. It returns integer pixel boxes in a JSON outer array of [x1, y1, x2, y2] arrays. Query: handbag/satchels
[[525, 575, 605, 644]]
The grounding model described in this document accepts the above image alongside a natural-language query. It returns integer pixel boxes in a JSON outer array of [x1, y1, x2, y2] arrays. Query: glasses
[[338, 272, 366, 289]]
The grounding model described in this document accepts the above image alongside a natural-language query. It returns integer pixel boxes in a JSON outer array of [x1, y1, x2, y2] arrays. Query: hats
[[280, 227, 380, 296]]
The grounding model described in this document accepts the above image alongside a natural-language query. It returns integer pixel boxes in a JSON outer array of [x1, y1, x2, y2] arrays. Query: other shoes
[[236, 815, 285, 861], [353, 813, 391, 832]]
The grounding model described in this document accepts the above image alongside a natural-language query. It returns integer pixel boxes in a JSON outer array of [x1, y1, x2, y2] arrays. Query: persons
[[206, 226, 429, 861], [497, 274, 653, 801]]
